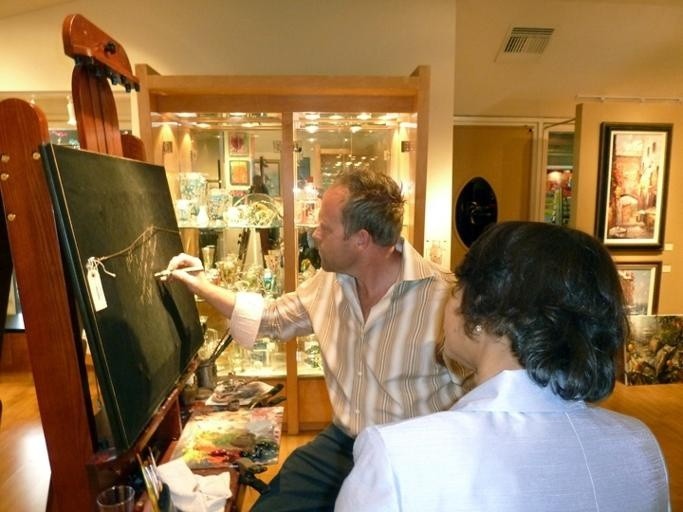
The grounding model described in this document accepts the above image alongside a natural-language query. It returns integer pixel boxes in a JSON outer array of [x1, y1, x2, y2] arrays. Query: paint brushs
[[241, 375, 258, 386], [208, 327, 234, 362]]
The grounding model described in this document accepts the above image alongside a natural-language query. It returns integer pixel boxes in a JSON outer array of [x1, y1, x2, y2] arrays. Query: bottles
[[299, 176, 317, 225]]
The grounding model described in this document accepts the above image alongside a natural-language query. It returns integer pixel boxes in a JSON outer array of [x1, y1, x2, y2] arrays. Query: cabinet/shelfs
[[131, 64, 432, 431]]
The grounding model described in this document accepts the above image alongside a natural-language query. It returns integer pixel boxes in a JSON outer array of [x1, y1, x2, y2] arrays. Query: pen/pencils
[[154, 266, 204, 277], [135, 446, 163, 512]]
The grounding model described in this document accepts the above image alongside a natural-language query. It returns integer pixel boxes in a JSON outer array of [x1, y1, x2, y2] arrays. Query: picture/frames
[[607, 260, 663, 315], [590, 119, 675, 258]]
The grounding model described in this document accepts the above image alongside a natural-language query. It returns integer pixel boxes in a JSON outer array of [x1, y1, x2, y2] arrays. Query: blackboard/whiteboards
[[38, 141, 204, 451]]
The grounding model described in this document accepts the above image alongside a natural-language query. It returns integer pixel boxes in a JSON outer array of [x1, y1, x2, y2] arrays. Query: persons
[[160, 167, 478, 512], [332, 220, 671, 512]]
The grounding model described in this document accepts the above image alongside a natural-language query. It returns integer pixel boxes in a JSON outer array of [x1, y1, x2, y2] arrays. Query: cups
[[174, 170, 226, 226], [183, 383, 197, 405], [95, 485, 133, 510], [195, 359, 218, 390]]
